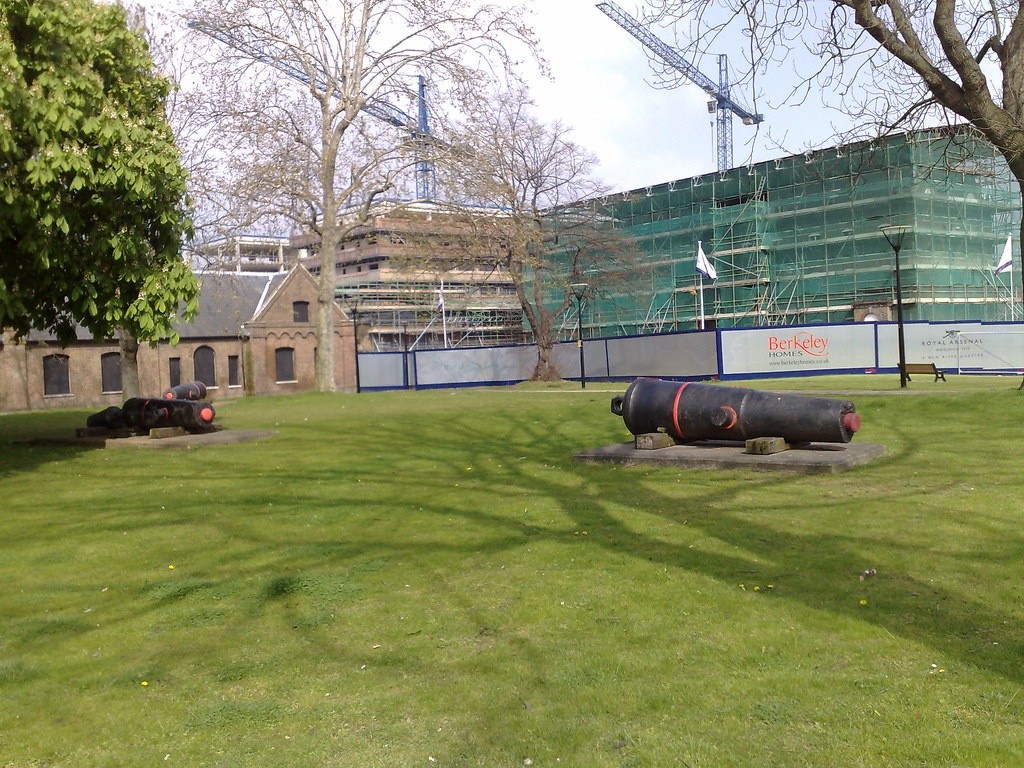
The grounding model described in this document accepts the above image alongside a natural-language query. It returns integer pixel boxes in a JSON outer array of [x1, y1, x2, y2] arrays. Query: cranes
[[186, 16, 476, 201], [595, 1, 766, 175]]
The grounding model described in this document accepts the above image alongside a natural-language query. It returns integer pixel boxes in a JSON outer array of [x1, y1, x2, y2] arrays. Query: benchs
[[897, 363, 947, 382]]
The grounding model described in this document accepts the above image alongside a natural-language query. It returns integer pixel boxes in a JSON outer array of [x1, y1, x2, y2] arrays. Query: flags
[[696, 244, 719, 280], [994, 235, 1012, 276], [437, 288, 442, 312]]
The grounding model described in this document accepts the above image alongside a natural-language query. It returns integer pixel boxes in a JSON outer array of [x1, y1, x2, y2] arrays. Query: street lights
[[569, 282, 590, 388], [879, 224, 914, 388], [344, 297, 361, 393]]
